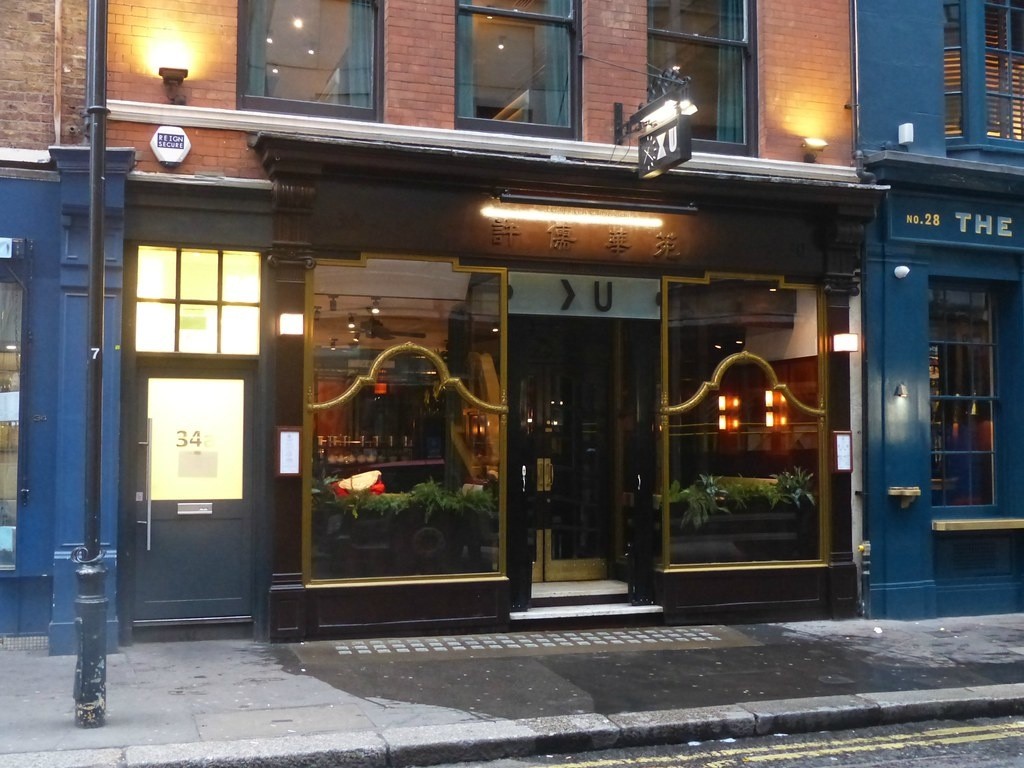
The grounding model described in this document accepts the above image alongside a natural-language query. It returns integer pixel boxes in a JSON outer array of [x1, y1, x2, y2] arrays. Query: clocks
[[640, 118, 678, 174]]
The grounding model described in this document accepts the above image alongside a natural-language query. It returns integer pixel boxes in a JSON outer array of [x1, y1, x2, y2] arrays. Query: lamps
[[347, 313, 355, 328], [313, 305, 322, 320], [801, 138, 828, 162], [896, 385, 909, 398], [274, 426, 302, 477], [353, 331, 360, 341], [765, 389, 774, 427], [636, 97, 699, 132], [718, 395, 726, 431], [833, 332, 859, 352], [371, 296, 381, 313], [329, 337, 337, 351], [831, 431, 853, 473], [328, 295, 339, 311], [159, 68, 187, 99], [276, 310, 303, 338]]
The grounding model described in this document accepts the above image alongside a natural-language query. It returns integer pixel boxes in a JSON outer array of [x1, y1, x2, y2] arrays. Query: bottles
[[357, 435, 386, 464], [401, 436, 409, 461], [387, 435, 398, 462], [318, 436, 356, 465]]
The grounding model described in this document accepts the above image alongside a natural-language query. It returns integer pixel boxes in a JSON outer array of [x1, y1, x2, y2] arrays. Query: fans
[[359, 306, 425, 340]]
